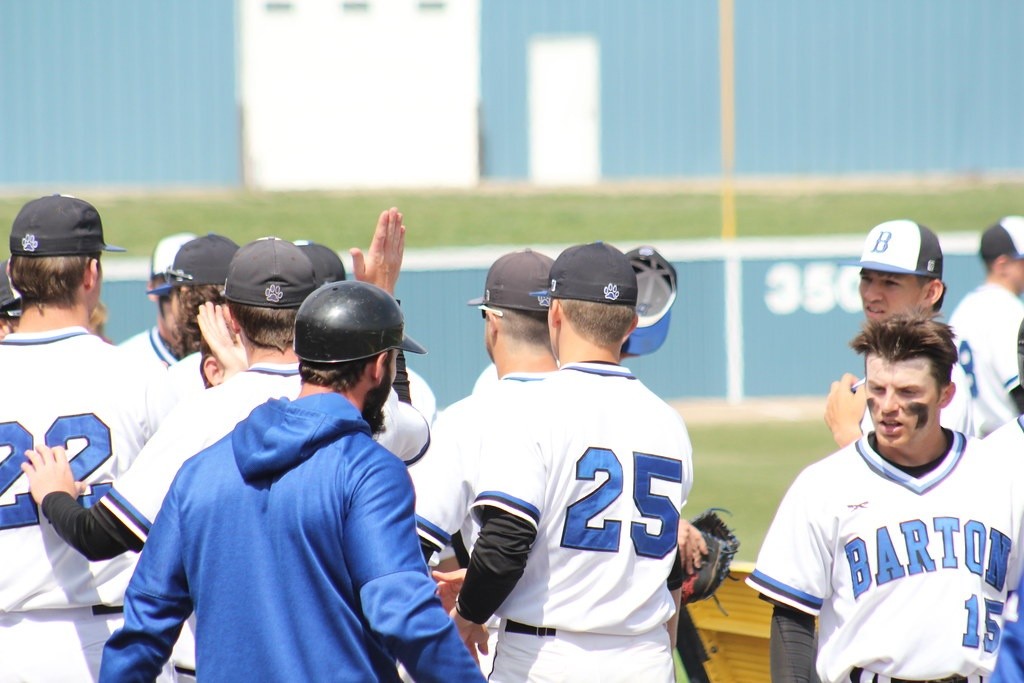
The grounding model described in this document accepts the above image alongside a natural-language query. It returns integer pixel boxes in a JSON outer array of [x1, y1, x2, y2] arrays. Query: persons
[[744, 315, 1024, 683], [951, 215, 1024, 415], [823, 219, 975, 451], [0, 193, 707, 683]]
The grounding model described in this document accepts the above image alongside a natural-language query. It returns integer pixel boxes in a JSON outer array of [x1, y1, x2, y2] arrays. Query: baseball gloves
[[681, 512, 740, 606]]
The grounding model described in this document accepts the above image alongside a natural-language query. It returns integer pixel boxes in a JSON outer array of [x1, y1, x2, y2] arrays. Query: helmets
[[293, 280, 426, 362]]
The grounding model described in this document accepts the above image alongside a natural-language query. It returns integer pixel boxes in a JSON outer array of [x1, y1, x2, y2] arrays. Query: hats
[[9, 194, 126, 257], [621, 245, 677, 355], [0, 262, 24, 311], [981, 215, 1024, 258], [151, 234, 196, 278], [527, 242, 639, 307], [222, 237, 318, 307], [853, 218, 943, 278], [148, 234, 240, 294], [293, 240, 346, 287], [467, 249, 558, 309]]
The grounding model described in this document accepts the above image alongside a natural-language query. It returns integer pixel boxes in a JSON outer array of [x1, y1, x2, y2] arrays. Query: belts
[[93, 603, 125, 614], [174, 666, 196, 676], [505, 619, 555, 636], [850, 668, 982, 683]]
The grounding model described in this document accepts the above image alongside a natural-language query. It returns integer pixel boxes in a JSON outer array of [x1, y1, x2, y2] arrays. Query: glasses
[[478, 303, 502, 319], [163, 266, 194, 280]]
